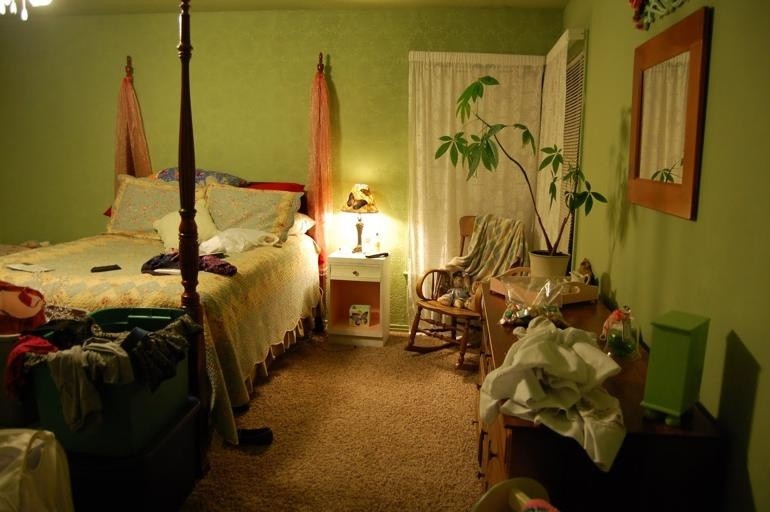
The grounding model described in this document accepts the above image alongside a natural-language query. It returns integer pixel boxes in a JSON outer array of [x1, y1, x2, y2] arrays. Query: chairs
[[404, 214, 527, 372]]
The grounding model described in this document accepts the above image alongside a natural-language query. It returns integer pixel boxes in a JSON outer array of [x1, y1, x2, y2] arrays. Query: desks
[[472, 274, 728, 511]]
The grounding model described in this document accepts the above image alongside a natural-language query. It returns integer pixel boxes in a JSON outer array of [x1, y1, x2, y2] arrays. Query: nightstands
[[325, 250, 392, 347]]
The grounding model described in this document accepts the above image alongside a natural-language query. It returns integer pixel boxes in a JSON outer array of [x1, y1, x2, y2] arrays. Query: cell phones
[[91, 264, 121, 272]]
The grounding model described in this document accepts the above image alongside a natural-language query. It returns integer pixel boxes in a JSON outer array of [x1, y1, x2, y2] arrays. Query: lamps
[[340, 184, 379, 253]]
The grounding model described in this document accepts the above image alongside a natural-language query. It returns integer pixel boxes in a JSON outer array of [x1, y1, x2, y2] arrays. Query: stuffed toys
[[436, 271, 485, 309]]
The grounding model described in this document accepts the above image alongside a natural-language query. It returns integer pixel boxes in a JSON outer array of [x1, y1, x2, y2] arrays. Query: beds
[[0, 232, 319, 478]]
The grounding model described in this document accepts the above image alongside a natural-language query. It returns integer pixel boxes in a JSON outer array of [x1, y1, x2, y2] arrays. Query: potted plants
[[433, 75, 607, 279]]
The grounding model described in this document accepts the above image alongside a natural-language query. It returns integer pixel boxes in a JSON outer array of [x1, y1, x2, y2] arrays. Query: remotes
[[153, 266, 181, 275], [366, 253, 388, 258]]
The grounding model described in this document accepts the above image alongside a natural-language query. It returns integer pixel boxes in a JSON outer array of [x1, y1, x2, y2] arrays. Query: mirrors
[[626, 6, 714, 222]]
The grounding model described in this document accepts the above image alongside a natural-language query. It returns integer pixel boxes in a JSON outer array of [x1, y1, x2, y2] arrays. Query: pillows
[[106, 166, 316, 249]]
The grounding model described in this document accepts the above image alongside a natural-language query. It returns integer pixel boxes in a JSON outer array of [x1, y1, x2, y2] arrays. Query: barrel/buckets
[[529, 249, 570, 276]]
